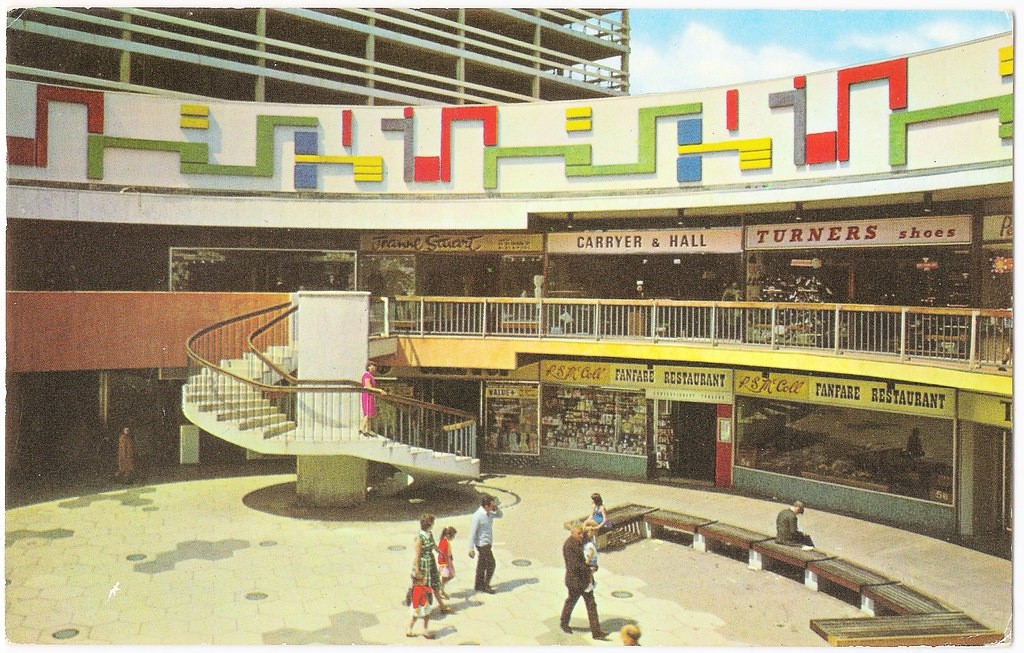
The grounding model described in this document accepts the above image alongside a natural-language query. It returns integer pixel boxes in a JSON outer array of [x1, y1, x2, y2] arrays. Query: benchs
[[810, 614, 1004, 647], [565, 503, 964, 615]]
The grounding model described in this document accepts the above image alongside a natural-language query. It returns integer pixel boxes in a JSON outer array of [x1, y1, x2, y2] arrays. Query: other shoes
[[406, 632, 417, 637], [593, 632, 610, 638], [423, 633, 435, 639], [585, 583, 594, 592], [440, 606, 452, 613], [561, 622, 573, 634], [441, 591, 450, 599], [359, 430, 373, 437], [475, 585, 495, 594]]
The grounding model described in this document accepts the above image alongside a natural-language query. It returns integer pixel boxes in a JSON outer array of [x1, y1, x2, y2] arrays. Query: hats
[[794, 500, 805, 515]]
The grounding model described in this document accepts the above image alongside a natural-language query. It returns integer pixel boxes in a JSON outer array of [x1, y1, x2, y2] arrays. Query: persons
[[437, 527, 457, 600], [468, 495, 503, 593], [405, 570, 434, 639], [414, 514, 452, 614], [775, 501, 815, 548], [582, 531, 598, 592], [561, 525, 608, 639], [358, 361, 387, 437], [998, 308, 1014, 371], [621, 624, 641, 646], [584, 493, 607, 529]]
